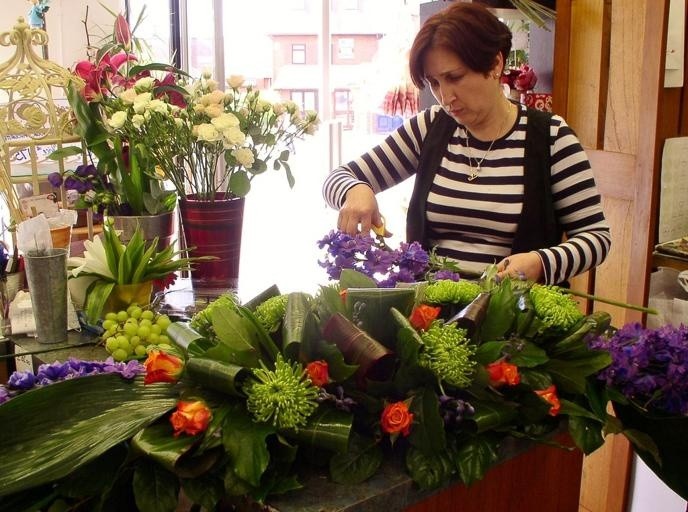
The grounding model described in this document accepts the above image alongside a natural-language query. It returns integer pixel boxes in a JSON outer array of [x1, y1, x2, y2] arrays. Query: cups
[[0, 270, 26, 318]]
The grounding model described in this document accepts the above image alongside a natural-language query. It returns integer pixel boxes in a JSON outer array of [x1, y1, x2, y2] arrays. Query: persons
[[320, 1, 615, 302]]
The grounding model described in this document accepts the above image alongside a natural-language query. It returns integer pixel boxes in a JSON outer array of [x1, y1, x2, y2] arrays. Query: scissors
[[337, 213, 393, 252]]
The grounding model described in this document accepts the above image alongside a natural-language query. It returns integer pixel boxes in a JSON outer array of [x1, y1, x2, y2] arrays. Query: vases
[[178, 191, 245, 283], [107, 210, 175, 254], [104, 284, 154, 314]]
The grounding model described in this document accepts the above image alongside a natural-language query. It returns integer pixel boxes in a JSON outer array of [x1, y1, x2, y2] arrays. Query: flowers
[[108, 70, 319, 199], [322, 231, 660, 315], [1, 342, 189, 509], [67, 218, 220, 283], [189, 284, 477, 512], [470, 259, 687, 511], [49, 13, 191, 213]]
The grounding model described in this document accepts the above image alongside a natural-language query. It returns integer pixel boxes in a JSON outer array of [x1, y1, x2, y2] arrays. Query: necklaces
[[465, 99, 511, 182]]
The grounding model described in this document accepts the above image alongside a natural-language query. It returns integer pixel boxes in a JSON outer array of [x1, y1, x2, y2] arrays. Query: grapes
[[92, 304, 172, 363]]
